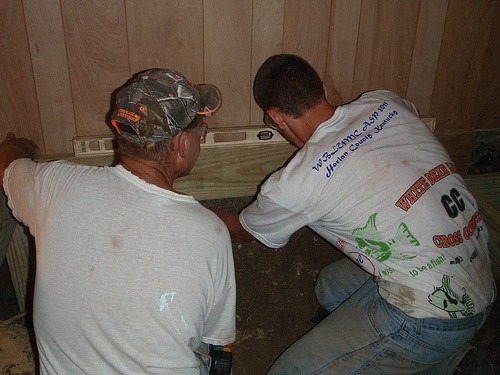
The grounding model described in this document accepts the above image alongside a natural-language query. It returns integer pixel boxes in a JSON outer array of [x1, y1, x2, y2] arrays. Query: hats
[[107, 67, 223, 143]]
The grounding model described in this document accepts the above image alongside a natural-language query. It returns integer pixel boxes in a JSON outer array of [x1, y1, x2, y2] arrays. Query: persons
[[0, 67, 236, 375], [208, 54, 496, 375]]
